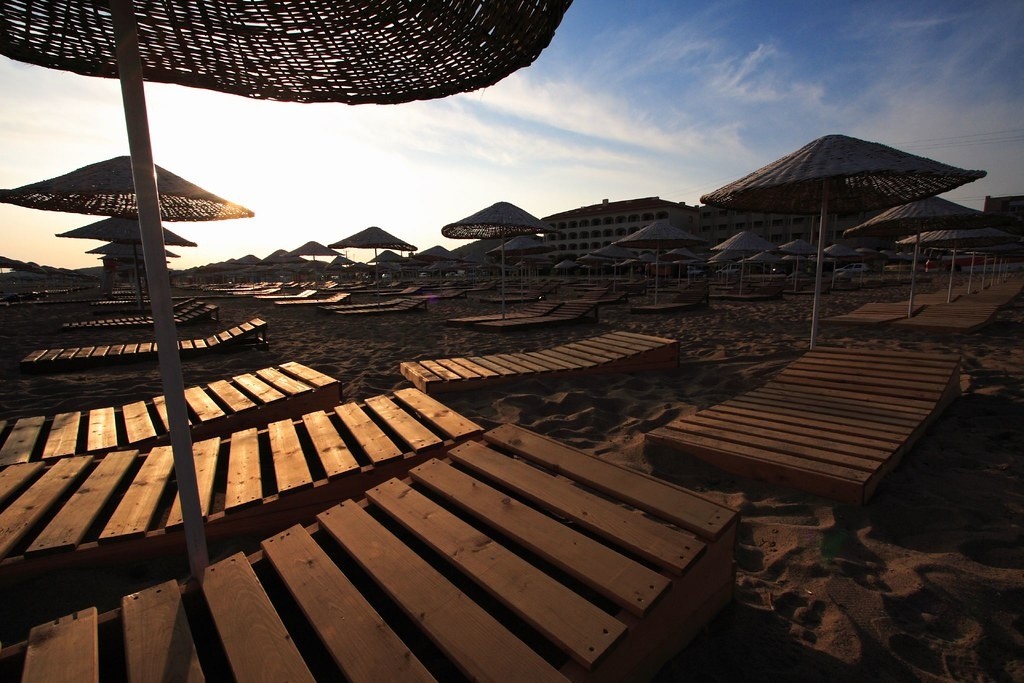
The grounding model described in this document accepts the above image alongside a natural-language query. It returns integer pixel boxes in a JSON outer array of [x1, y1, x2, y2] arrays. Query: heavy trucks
[[644, 262, 706, 278]]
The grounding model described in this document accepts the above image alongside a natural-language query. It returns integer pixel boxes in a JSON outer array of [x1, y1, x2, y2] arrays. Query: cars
[[445, 271, 458, 277], [833, 262, 871, 275], [418, 273, 432, 279], [0, 291, 51, 304]]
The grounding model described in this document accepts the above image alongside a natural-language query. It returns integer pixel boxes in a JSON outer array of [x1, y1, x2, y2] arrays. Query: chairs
[[0, 270, 1024, 683]]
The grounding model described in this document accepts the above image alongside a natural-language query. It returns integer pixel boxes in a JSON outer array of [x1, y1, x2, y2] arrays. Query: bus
[[923, 246, 1024, 274]]
